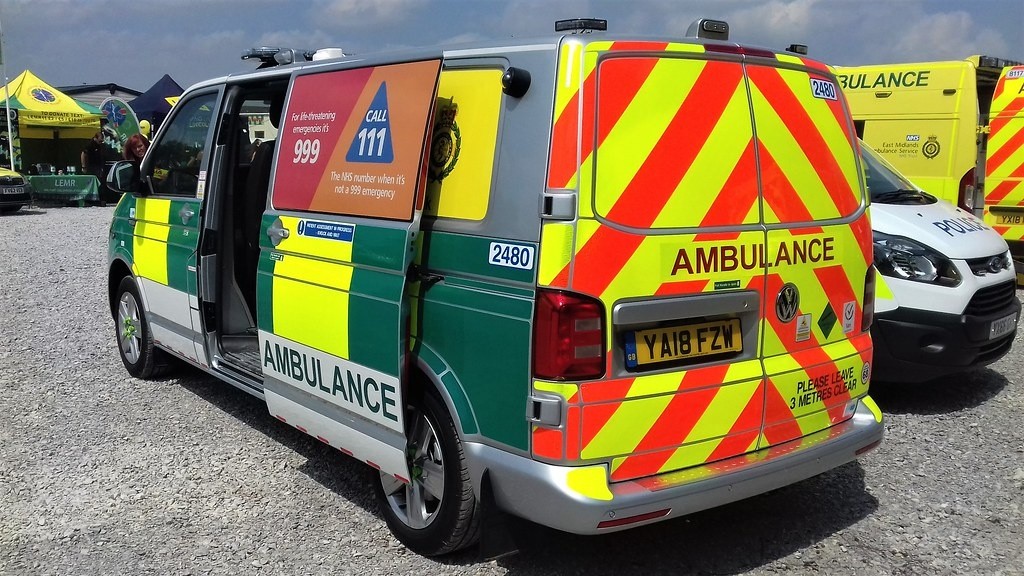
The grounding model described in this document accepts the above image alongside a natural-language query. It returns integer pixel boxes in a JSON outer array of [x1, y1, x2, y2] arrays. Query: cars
[[0, 168, 32, 212]]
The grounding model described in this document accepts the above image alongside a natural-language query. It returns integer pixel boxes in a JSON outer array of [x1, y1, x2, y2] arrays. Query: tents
[[0, 69, 214, 173]]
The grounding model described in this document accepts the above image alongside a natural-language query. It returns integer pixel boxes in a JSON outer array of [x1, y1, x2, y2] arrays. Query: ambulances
[[831, 55, 1024, 285], [107, 16, 884, 547]]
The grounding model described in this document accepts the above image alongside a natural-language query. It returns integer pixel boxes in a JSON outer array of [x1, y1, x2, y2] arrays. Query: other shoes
[[91, 202, 100, 206]]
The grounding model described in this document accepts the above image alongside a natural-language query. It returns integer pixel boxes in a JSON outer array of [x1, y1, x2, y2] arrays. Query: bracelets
[[82, 167, 87, 170]]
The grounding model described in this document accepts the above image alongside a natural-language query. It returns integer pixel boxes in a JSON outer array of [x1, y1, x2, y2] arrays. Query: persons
[[123, 132, 170, 178], [81, 134, 107, 207]]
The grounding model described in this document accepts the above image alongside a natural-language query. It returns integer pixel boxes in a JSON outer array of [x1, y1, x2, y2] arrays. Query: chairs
[[236, 92, 286, 248]]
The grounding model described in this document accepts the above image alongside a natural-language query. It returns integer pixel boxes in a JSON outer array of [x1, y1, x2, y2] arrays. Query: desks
[[24, 175, 101, 209]]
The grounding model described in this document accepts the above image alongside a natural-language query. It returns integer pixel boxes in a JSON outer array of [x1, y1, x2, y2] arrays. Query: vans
[[857, 134, 1018, 375]]
